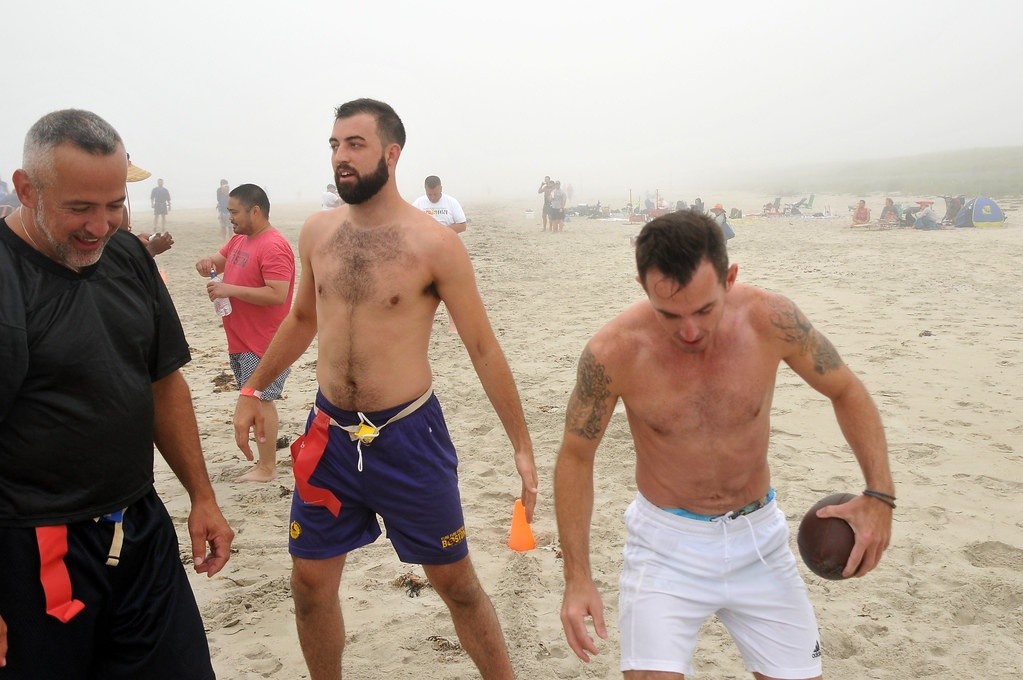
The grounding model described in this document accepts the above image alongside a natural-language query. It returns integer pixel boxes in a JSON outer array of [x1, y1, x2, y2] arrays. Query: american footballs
[[796, 492, 864, 581]]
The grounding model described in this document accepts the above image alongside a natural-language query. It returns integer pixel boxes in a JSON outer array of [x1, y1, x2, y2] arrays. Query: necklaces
[[19, 205, 78, 273]]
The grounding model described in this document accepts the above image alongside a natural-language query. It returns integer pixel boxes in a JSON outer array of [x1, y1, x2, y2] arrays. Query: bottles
[[210, 269, 233, 317]]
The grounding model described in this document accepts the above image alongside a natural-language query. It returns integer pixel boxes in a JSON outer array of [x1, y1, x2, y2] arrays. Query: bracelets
[[865, 489, 897, 500], [239, 388, 262, 399], [862, 492, 895, 509]]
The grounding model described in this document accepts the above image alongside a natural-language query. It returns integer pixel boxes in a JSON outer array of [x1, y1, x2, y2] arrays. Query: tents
[[956, 197, 1006, 228]]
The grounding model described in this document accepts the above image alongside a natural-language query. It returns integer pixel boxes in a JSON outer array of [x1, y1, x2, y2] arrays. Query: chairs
[[762, 194, 815, 215]]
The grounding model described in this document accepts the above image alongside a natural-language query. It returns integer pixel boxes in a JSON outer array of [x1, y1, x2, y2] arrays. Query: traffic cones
[[507, 499, 537, 550]]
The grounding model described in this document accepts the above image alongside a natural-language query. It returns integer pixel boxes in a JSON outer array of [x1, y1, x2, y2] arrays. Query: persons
[[194, 184, 295, 483], [0, 109, 235, 680], [553, 210, 896, 680], [0, 151, 965, 254], [233, 98, 538, 680]]
